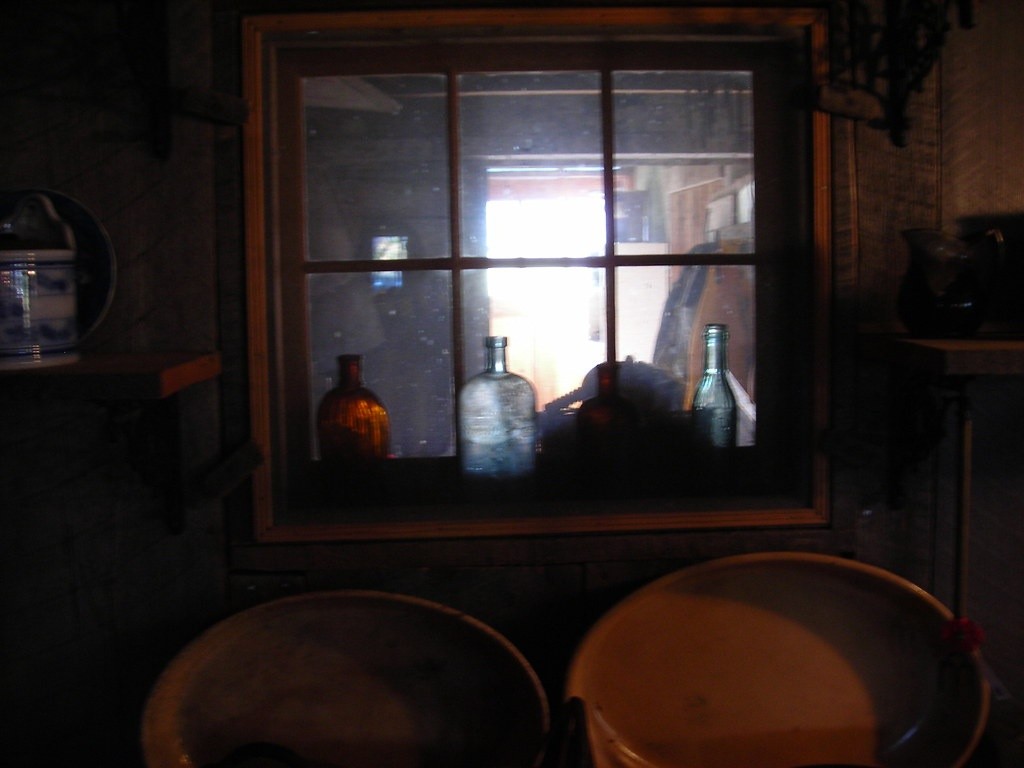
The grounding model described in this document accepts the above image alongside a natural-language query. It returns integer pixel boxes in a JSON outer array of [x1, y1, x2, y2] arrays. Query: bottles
[[691, 324, 735, 493], [577, 364, 639, 497], [317, 354, 390, 500], [455, 336, 537, 482], [0, 194, 80, 370]]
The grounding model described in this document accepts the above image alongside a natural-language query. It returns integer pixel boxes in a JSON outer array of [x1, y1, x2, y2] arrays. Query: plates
[[560, 552, 991, 768], [0, 188, 117, 342], [140, 591, 551, 768]]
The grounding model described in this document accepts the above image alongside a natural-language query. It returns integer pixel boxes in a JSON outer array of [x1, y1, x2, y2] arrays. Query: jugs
[[898, 229, 1004, 335]]
